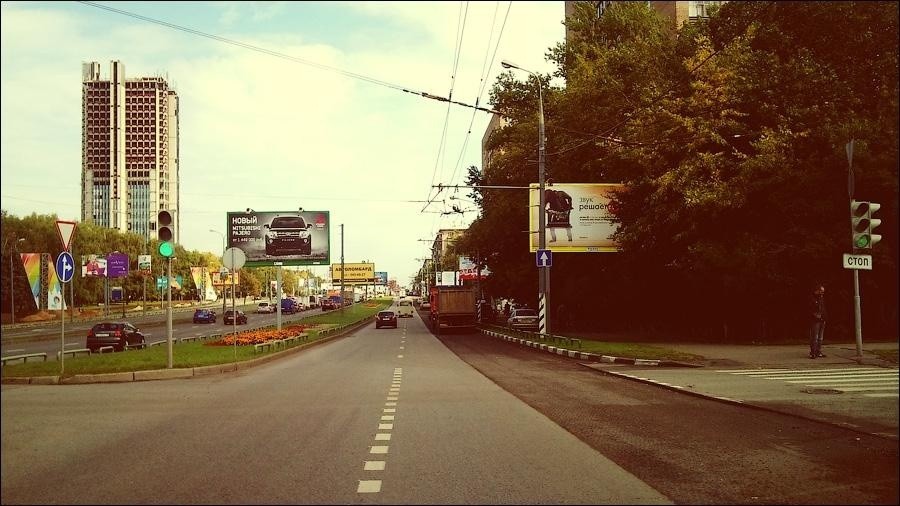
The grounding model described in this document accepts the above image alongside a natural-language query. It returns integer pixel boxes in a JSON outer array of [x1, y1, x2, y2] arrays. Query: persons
[[545, 188, 572, 243], [806, 284, 828, 360], [504, 301, 510, 310]]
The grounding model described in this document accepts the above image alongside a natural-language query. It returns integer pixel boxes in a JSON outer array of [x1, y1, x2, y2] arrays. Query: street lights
[[450, 197, 482, 323], [499, 60, 550, 339], [210, 229, 227, 313], [9, 238, 27, 325]]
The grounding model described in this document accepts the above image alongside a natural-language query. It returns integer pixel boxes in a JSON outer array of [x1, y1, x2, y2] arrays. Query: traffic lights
[[854, 201, 871, 252], [158, 210, 176, 260], [871, 203, 881, 247]]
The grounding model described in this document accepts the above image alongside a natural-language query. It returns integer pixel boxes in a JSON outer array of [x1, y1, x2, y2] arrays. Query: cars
[[223, 309, 247, 325], [367, 294, 384, 298], [409, 291, 419, 296], [281, 295, 322, 314], [375, 311, 398, 329], [507, 308, 538, 333], [417, 297, 431, 310], [192, 309, 217, 325], [264, 216, 314, 256], [87, 322, 147, 355], [257, 303, 275, 313]]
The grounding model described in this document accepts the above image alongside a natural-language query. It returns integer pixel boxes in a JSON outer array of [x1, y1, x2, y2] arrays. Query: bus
[[397, 298, 414, 319], [405, 289, 409, 295]]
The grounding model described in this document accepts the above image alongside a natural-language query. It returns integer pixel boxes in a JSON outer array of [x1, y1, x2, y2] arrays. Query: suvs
[[322, 299, 336, 311]]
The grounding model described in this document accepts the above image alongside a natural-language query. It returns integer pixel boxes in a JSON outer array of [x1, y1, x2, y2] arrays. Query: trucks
[[399, 289, 406, 299], [330, 291, 364, 308], [428, 288, 477, 334]]
